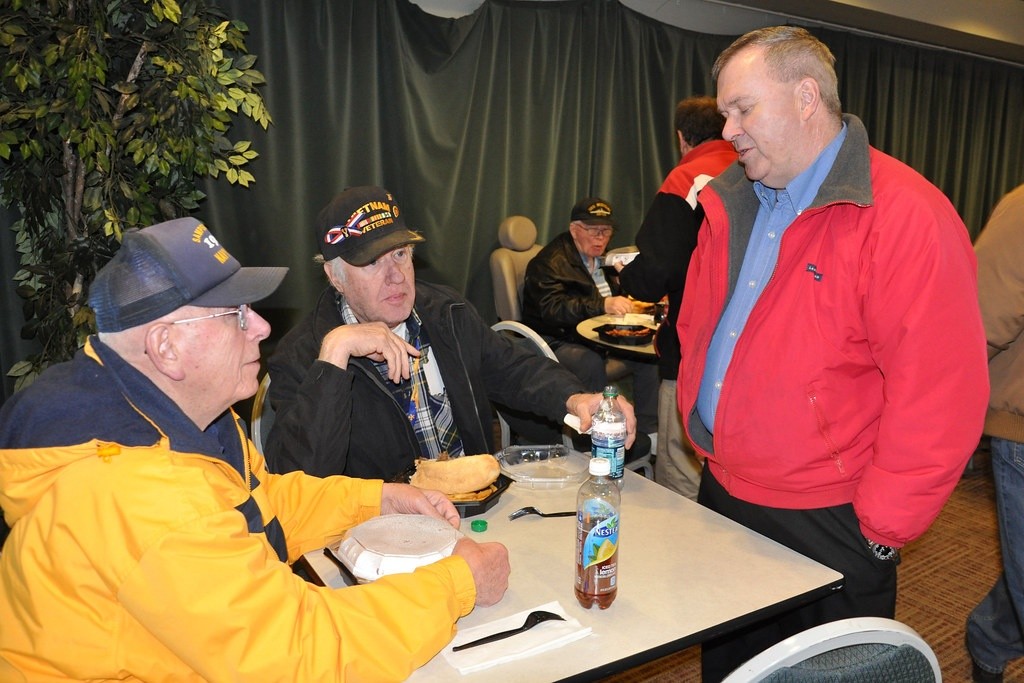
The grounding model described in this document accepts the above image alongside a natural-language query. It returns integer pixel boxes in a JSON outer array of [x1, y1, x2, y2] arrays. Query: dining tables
[[575, 300, 665, 367], [296, 450, 846, 683]]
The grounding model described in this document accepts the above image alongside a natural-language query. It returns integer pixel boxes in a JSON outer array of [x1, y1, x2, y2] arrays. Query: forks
[[507, 506, 577, 522], [453, 611, 567, 652]]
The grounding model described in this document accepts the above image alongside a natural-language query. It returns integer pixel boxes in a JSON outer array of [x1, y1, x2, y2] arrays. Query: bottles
[[575, 457, 620, 609], [591, 383, 624, 493]]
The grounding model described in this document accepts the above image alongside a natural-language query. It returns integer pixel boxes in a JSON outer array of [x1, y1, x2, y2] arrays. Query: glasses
[[143, 303, 255, 355], [575, 224, 615, 236]]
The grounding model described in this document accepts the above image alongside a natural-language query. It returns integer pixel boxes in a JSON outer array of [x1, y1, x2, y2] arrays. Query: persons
[[264, 184, 636, 483], [966, 184, 1024, 683], [0, 217, 510, 683], [610, 97, 739, 502], [677, 26, 990, 683], [523, 196, 661, 458]]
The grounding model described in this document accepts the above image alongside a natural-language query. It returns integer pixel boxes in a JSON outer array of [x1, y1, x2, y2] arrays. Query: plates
[[592, 323, 657, 346], [495, 446, 593, 486]]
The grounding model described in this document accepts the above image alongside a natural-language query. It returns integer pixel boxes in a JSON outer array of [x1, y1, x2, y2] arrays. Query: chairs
[[488, 214, 656, 486], [722, 617, 944, 683]]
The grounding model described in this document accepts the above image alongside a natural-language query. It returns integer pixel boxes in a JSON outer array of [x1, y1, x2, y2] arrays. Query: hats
[[570, 196, 617, 225], [87, 215, 290, 335], [315, 184, 427, 268]]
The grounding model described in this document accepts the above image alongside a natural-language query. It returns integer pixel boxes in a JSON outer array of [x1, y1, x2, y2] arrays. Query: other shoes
[[965, 635, 1004, 683]]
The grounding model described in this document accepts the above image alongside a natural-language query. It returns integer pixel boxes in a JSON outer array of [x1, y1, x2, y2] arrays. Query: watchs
[[867, 539, 896, 560]]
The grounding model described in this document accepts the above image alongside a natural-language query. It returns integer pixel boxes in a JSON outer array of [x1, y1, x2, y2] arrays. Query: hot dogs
[[407, 450, 501, 494]]
